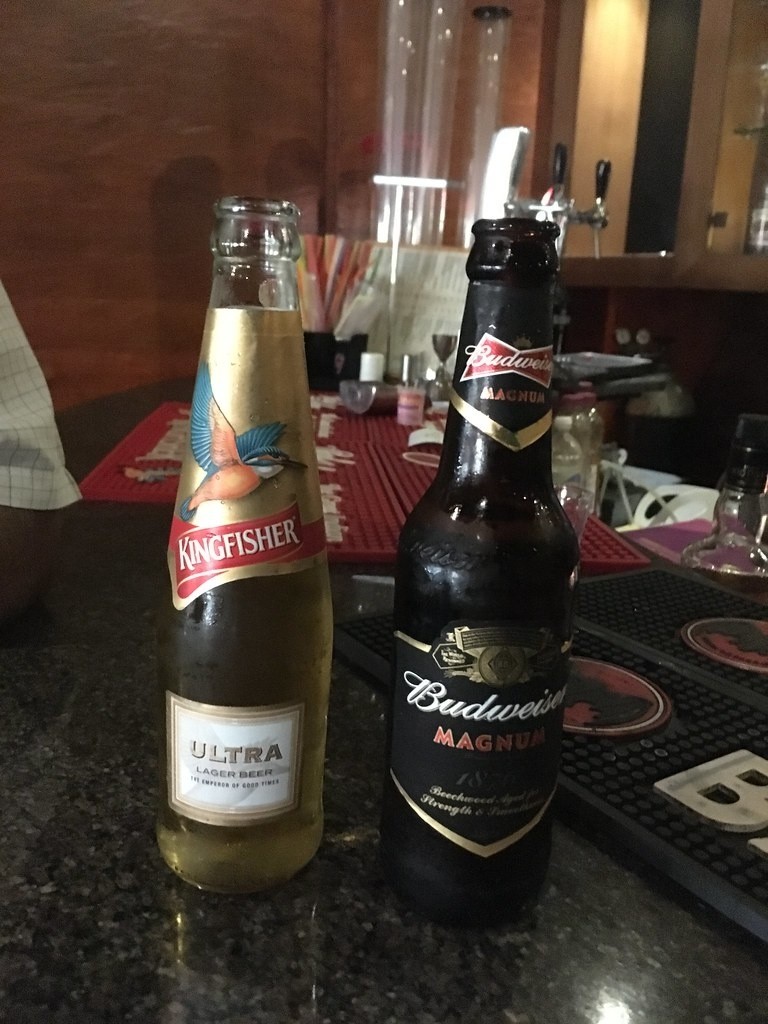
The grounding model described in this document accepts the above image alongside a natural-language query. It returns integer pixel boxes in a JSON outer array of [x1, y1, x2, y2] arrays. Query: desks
[[559, 253, 767, 457]]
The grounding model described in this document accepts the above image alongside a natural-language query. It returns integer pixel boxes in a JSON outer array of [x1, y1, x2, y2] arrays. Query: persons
[[0, 280, 85, 615]]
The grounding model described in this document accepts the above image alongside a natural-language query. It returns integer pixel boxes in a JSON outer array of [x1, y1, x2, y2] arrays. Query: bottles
[[155, 195, 333, 898], [379, 218, 581, 929], [549, 378, 604, 518]]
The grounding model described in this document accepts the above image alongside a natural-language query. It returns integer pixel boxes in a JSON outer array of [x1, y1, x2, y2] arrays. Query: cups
[[553, 485, 594, 546], [303, 331, 336, 391]]
